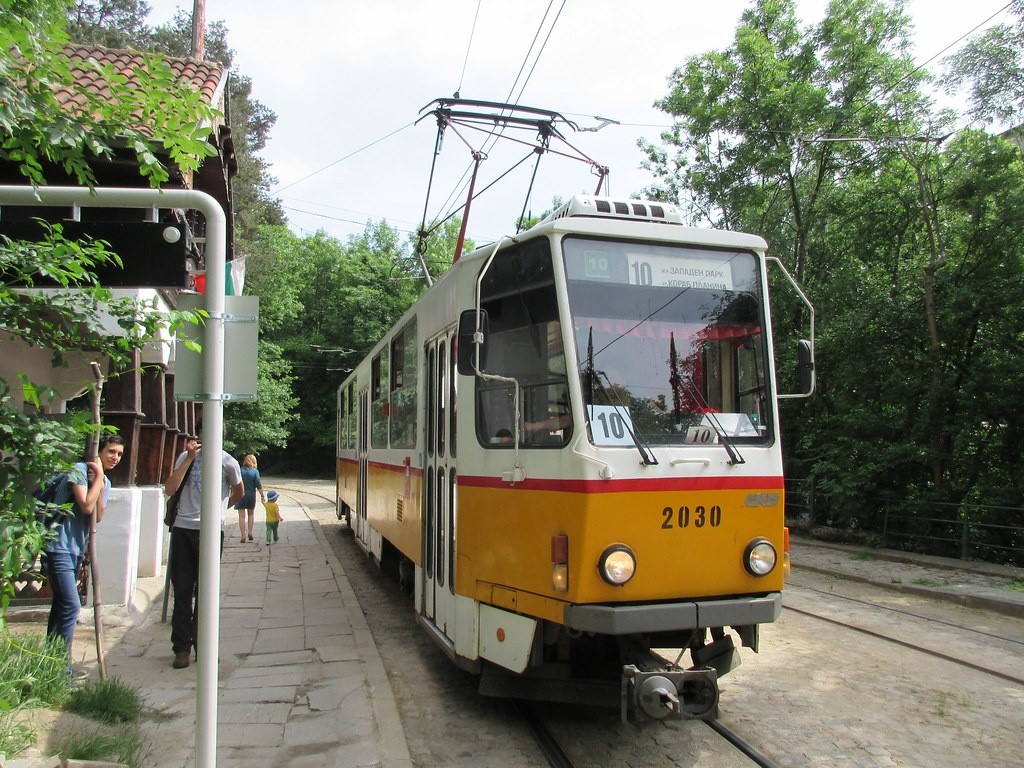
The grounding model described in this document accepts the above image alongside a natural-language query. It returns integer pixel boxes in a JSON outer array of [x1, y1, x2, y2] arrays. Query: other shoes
[[248, 533, 254, 540], [70, 670, 90, 680], [173, 646, 189, 669], [266, 541, 270, 545], [241, 537, 245, 543]]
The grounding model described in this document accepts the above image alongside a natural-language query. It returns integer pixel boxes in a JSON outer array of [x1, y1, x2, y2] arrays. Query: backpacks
[[31, 460, 89, 533]]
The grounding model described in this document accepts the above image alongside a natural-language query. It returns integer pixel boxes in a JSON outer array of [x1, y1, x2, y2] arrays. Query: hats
[[267, 489, 279, 501]]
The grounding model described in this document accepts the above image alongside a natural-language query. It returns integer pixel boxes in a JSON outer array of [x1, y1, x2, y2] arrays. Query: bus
[[337, 94, 818, 729]]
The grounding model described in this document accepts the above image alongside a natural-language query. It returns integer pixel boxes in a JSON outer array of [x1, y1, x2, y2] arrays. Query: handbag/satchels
[[164, 457, 195, 526]]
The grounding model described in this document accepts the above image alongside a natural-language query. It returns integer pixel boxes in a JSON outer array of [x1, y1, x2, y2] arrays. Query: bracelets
[[261, 496, 264, 497]]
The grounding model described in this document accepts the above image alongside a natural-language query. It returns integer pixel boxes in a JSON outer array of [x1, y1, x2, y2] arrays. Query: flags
[[195, 257, 245, 296]]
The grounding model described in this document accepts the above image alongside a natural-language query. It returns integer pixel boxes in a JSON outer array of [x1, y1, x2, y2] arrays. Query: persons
[[525, 366, 664, 432], [234, 454, 264, 543], [262, 491, 283, 546], [165, 417, 244, 667], [496, 429, 513, 443], [42, 434, 124, 679]]
